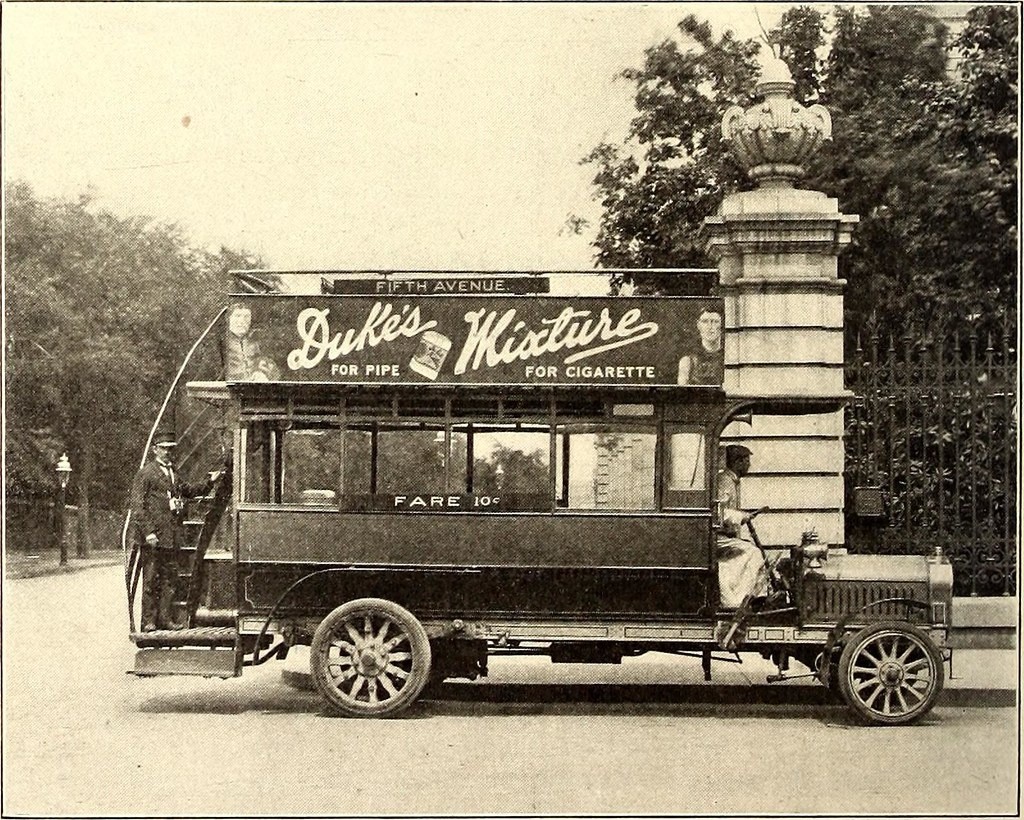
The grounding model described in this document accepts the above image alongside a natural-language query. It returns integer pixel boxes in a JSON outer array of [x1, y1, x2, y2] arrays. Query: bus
[[116, 268, 959, 723]]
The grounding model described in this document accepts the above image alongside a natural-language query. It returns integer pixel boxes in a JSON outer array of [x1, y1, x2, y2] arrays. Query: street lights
[[54, 452, 75, 569], [493, 463, 508, 513]]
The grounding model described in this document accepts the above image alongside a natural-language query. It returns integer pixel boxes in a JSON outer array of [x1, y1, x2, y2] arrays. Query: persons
[[130, 431, 223, 636], [675, 307, 726, 384], [711, 445, 771, 613], [226, 304, 268, 382]]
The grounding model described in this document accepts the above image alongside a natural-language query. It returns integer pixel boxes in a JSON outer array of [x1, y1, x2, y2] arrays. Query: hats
[[151, 432, 178, 447], [726, 445, 753, 459]]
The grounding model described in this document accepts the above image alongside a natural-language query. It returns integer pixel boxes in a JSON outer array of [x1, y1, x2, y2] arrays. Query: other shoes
[[162, 621, 183, 630], [145, 623, 156, 631]]
